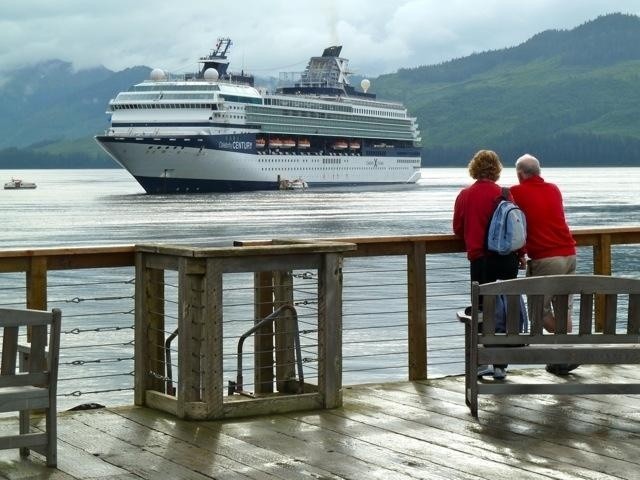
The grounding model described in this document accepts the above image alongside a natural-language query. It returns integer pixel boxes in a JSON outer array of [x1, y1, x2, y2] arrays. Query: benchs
[[455, 274, 639, 418], [0, 308, 62, 468]]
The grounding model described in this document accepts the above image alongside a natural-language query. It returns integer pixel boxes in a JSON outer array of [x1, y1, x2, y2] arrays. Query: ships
[[91, 30, 426, 195]]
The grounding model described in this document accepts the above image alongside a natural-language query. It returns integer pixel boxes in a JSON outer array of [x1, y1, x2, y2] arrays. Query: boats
[[3, 176, 38, 190]]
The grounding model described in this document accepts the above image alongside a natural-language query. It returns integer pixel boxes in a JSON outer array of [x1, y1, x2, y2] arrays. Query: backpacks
[[495, 279, 527, 333]]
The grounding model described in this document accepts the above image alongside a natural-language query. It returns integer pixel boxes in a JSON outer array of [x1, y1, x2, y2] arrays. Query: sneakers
[[546, 363, 580, 375], [476, 364, 506, 379]]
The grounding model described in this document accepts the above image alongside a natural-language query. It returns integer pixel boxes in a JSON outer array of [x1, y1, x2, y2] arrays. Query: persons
[[452, 149, 528, 381], [509, 153, 583, 376]]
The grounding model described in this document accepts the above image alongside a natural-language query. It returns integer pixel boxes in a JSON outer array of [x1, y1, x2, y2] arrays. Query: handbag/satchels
[[488, 188, 527, 255]]
